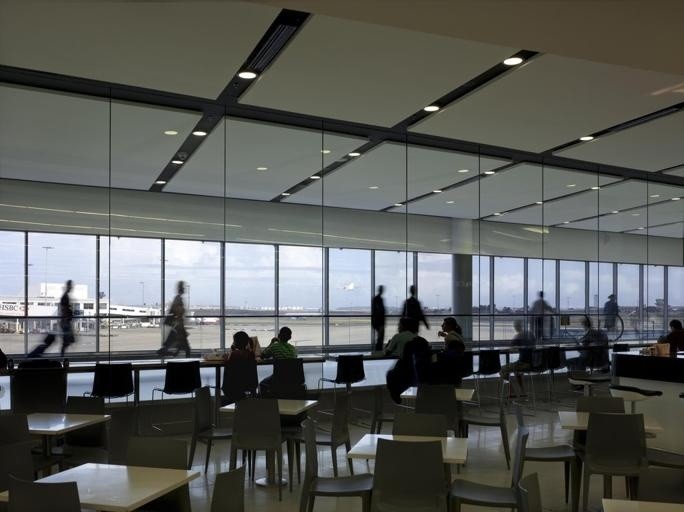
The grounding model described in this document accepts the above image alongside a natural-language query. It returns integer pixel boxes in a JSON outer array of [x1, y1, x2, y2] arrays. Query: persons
[[260, 326, 299, 398], [500, 321, 537, 397], [657, 319, 684, 352], [155, 278, 193, 359], [603, 294, 618, 335], [401, 285, 431, 337], [370, 285, 387, 352], [529, 290, 555, 338], [58, 277, 77, 355], [566, 317, 605, 392], [222, 329, 259, 400], [384, 317, 429, 404], [438, 318, 466, 353]]
